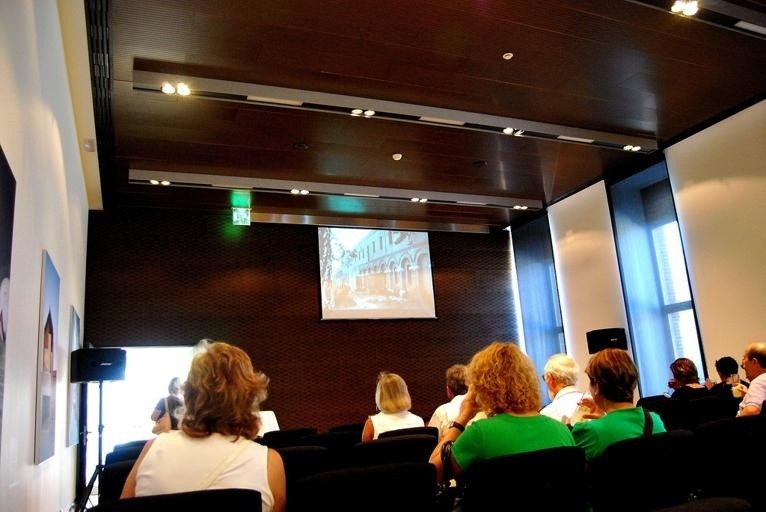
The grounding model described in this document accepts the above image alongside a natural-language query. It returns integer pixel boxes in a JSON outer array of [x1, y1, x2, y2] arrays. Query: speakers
[[587, 328, 627, 354], [71, 349, 125, 384]]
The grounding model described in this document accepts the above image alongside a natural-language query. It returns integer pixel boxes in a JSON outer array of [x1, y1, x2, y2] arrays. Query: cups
[[730, 371, 742, 398], [570, 386, 592, 427]]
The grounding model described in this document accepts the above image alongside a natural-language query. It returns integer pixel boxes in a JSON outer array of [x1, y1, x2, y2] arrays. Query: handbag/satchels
[[436, 440, 467, 512], [152, 398, 172, 435]]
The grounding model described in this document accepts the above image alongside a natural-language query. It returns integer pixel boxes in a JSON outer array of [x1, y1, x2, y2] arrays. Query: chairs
[[461, 446, 586, 511], [254, 423, 438, 465], [89, 488, 262, 512], [635, 389, 764, 432], [98, 442, 156, 503], [286, 462, 438, 510], [586, 431, 694, 511], [691, 413, 764, 511]]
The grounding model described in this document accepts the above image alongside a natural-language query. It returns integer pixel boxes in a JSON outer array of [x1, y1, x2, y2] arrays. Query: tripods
[[75, 380, 105, 512]]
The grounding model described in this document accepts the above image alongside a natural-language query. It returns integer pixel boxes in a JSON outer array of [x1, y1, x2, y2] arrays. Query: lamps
[[133, 70, 657, 155], [128, 168, 543, 212], [628, 0, 766, 41]]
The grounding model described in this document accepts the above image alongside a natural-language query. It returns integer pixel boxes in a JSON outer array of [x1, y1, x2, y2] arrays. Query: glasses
[[542, 374, 551, 381]]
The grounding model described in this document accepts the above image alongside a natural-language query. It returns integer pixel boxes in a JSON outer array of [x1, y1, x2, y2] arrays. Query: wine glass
[[664, 377, 676, 397]]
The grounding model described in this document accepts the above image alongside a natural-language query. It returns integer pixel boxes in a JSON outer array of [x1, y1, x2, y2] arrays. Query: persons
[[118, 340, 288, 512], [425, 341, 575, 482], [427, 362, 487, 429], [571, 347, 669, 461], [710, 356, 748, 395], [670, 358, 710, 399], [736, 342, 766, 417], [150, 377, 184, 431], [538, 353, 605, 425], [361, 372, 424, 445]]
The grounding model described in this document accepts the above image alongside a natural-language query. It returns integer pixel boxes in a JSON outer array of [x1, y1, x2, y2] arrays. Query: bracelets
[[447, 420, 466, 433]]
